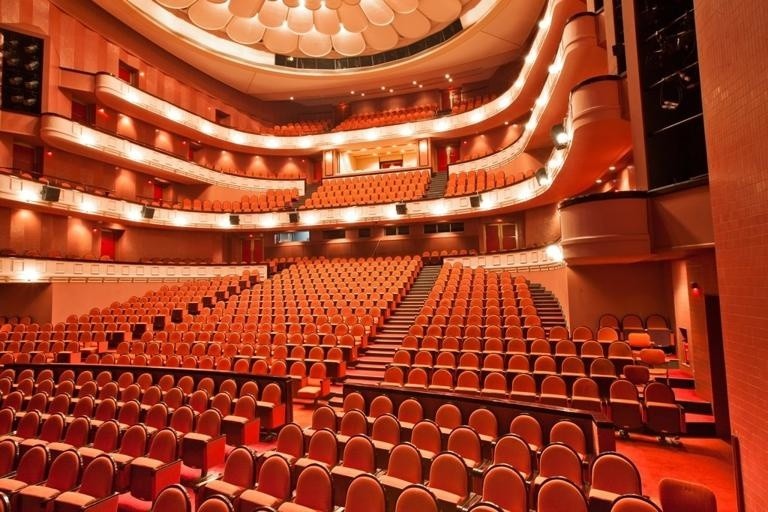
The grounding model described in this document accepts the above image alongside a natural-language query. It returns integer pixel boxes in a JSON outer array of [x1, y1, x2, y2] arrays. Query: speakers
[[42, 185, 60, 202], [470, 196, 481, 207], [535, 168, 547, 186], [550, 124, 567, 149], [396, 203, 406, 214], [288, 212, 299, 222], [230, 215, 240, 226], [142, 207, 155, 218]]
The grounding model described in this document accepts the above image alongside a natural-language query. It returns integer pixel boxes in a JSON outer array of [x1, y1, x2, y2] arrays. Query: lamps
[[550, 117, 571, 150]]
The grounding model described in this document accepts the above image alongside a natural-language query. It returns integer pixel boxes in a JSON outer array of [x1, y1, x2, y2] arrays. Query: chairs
[[0, 240, 730, 510], [0, 90, 545, 216]]
